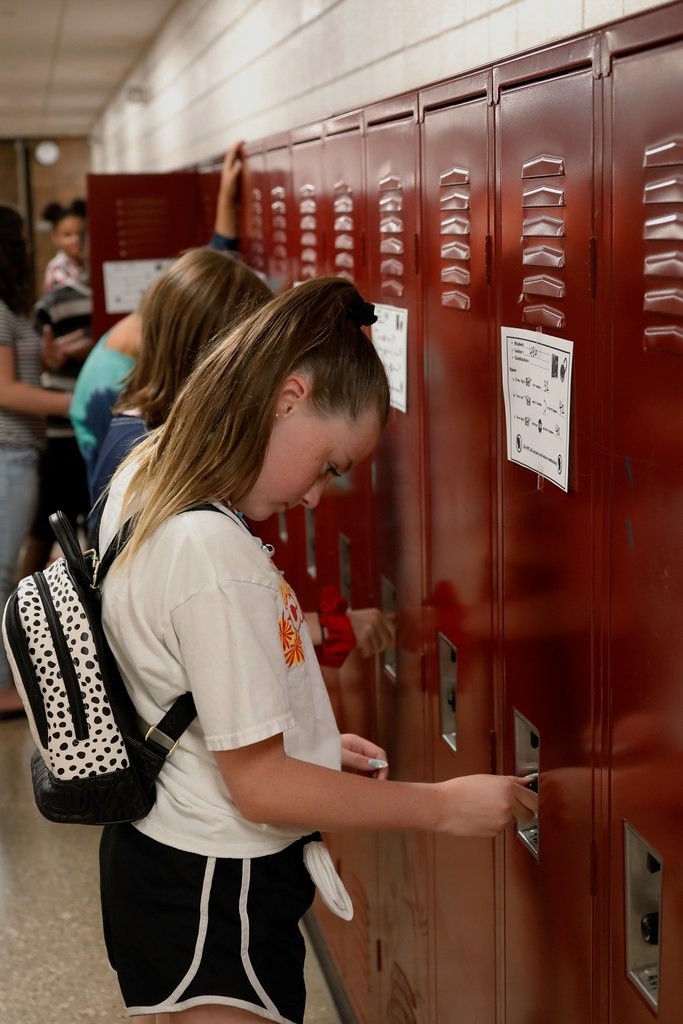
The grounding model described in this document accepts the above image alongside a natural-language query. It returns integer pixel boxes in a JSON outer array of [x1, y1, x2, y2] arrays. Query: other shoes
[[0, 708, 30, 721]]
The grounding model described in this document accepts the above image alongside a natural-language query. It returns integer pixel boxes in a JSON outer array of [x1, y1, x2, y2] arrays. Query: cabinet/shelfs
[[82, 0, 683, 1023]]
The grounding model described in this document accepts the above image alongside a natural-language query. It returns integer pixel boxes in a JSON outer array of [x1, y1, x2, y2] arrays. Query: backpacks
[[0, 482, 248, 825]]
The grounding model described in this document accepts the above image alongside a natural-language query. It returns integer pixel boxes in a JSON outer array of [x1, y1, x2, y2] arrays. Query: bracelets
[[317, 611, 326, 641], [210, 229, 240, 251]]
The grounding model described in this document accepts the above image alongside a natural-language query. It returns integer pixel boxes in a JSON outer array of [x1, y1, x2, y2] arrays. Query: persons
[[96, 277, 538, 1024], [0, 140, 276, 722]]
